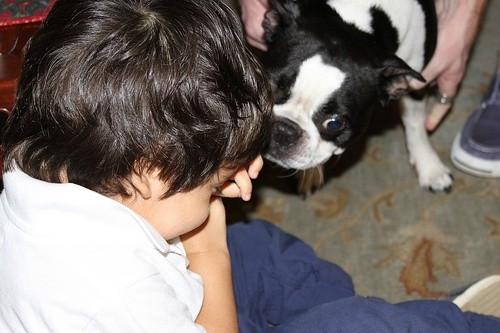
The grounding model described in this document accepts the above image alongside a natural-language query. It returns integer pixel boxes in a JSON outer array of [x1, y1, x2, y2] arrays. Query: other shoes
[[450, 44, 499, 177], [442, 273, 500, 318]]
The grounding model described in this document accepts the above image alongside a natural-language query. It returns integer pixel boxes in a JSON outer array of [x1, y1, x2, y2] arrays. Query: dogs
[[259, 0, 456, 196]]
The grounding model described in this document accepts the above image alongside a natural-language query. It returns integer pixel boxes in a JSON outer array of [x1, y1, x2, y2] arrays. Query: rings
[[435, 92, 454, 105]]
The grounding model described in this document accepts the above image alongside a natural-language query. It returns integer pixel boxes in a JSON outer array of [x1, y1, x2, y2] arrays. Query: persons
[[238, 0, 500, 178], [0, 0, 500, 333]]
